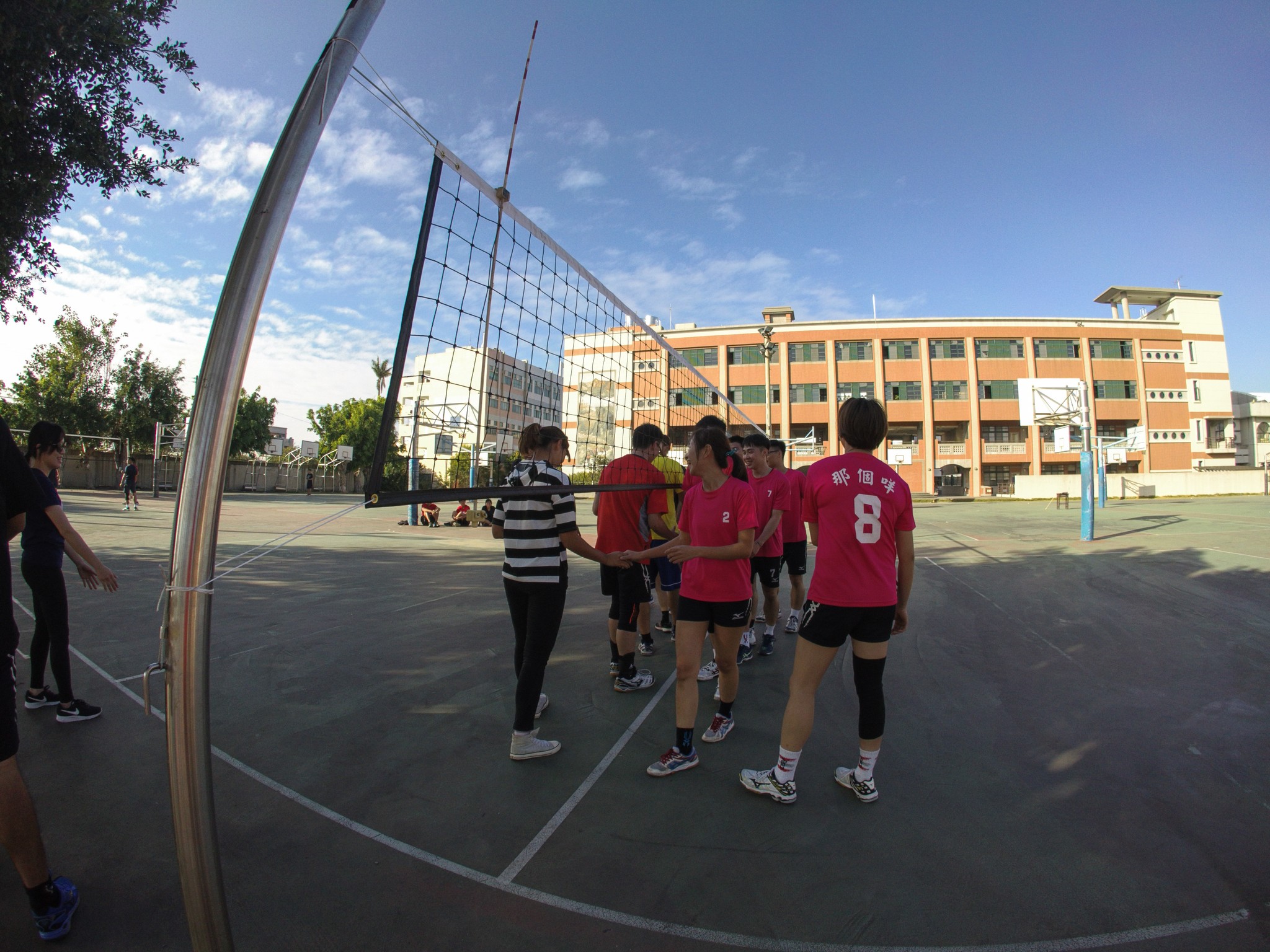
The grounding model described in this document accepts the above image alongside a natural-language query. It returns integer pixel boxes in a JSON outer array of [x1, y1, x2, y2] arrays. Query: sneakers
[[739, 766, 797, 803], [31, 876, 80, 940], [697, 659, 719, 679], [748, 627, 757, 645], [713, 678, 721, 701], [754, 609, 782, 623], [758, 633, 775, 655], [834, 766, 879, 803], [510, 727, 561, 760], [24, 685, 60, 709], [55, 699, 102, 722], [534, 693, 549, 719], [610, 658, 620, 675], [670, 626, 676, 641], [702, 710, 734, 742], [638, 638, 654, 656], [614, 668, 656, 693], [655, 621, 672, 631], [785, 616, 798, 633], [647, 746, 699, 776], [737, 644, 753, 665]]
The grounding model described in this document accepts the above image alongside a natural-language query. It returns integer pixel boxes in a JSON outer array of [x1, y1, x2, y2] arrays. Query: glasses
[[652, 442, 661, 453]]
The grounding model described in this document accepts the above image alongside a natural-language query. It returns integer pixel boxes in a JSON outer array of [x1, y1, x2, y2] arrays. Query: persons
[[444, 500, 471, 527], [492, 423, 633, 760], [306, 469, 314, 496], [19, 420, 120, 723], [0, 416, 79, 942], [593, 415, 807, 777], [478, 498, 495, 526], [118, 457, 141, 511], [420, 502, 440, 527], [737, 398, 916, 803]]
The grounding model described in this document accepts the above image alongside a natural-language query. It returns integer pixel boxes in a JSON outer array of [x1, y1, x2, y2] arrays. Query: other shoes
[[122, 501, 139, 511], [428, 521, 461, 527]]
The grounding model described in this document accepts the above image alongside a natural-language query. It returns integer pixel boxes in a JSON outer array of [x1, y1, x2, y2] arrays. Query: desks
[[1057, 493, 1069, 509]]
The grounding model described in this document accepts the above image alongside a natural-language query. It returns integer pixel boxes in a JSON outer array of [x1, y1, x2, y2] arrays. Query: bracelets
[[755, 540, 762, 546]]
